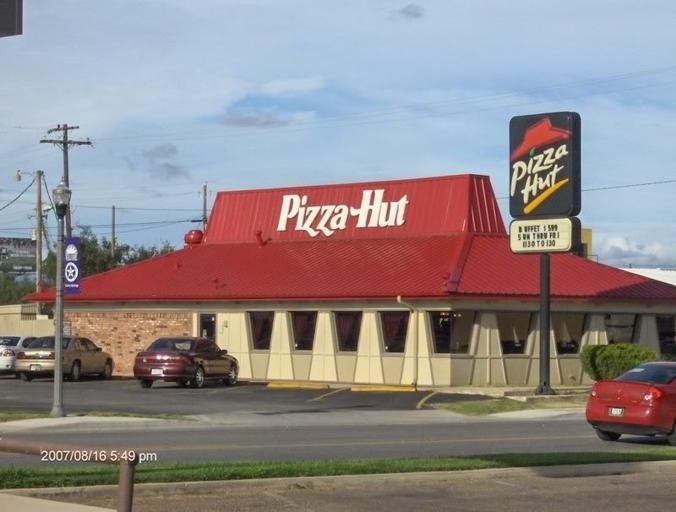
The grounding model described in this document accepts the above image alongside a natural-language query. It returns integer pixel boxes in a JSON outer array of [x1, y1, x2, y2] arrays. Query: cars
[[13, 335, 113, 381], [0, 336, 39, 375], [583, 361, 676, 446], [133, 337, 239, 388]]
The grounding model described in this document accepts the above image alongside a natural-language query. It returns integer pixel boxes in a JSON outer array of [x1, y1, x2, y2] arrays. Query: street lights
[[48, 177, 72, 421], [13, 168, 43, 314]]
